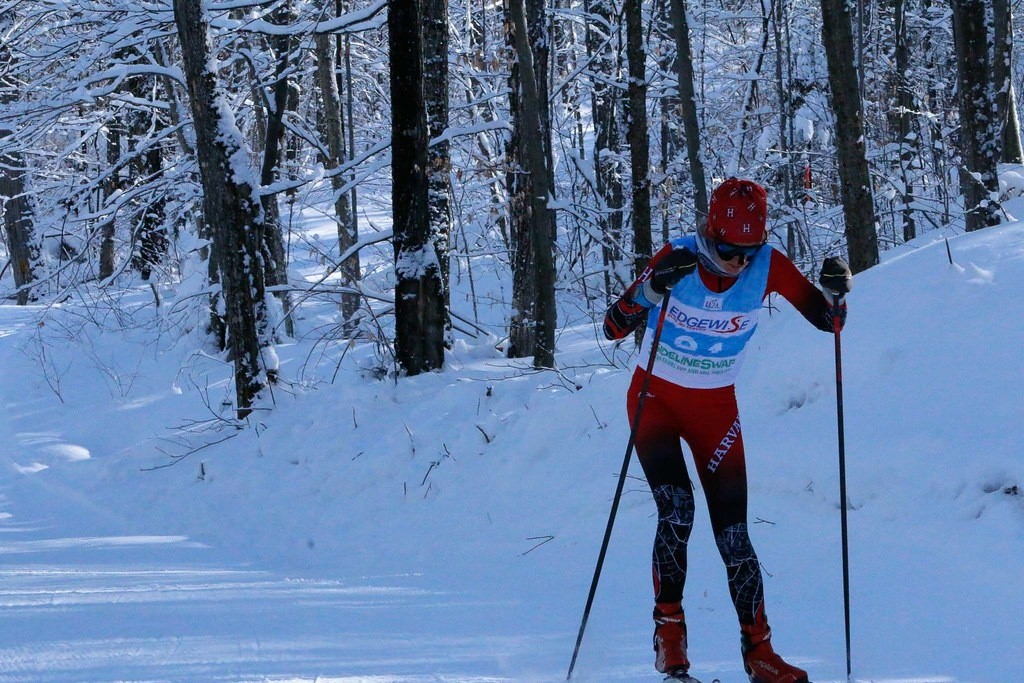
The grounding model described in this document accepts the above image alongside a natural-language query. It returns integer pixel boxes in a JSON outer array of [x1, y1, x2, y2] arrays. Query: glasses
[[712, 230, 770, 266]]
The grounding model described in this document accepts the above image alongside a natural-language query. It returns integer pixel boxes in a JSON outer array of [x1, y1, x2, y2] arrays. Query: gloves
[[649, 247, 698, 294], [819, 255, 852, 298]]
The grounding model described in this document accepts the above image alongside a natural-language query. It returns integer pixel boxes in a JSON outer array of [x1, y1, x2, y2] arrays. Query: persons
[[603, 177, 853, 683]]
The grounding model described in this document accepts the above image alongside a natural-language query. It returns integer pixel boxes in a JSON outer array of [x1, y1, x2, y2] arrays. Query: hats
[[704, 176, 767, 243]]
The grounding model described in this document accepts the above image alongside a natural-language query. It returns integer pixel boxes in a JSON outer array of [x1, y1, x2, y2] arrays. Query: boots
[[738, 617, 808, 683], [652, 604, 690, 678]]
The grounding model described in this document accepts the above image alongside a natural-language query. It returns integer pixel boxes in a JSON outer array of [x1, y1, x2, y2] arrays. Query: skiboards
[[661, 669, 815, 683]]
